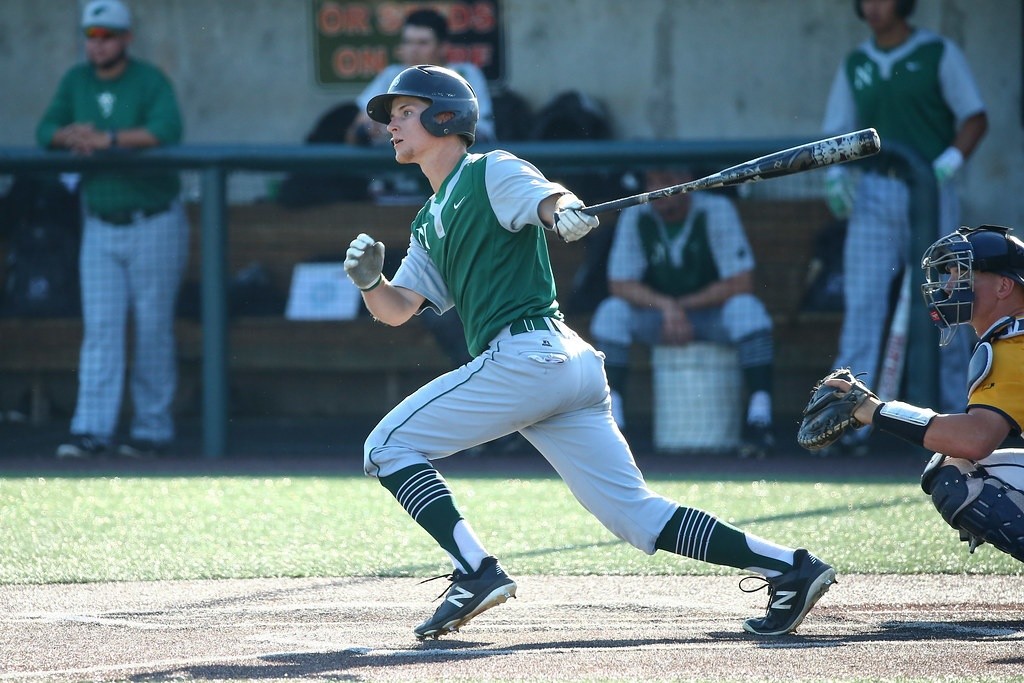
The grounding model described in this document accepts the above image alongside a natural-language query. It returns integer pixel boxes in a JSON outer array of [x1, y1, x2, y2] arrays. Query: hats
[[82, 0, 130, 31]]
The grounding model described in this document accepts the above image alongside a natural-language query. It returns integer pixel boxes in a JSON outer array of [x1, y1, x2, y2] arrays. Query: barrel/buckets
[[649, 339, 747, 454]]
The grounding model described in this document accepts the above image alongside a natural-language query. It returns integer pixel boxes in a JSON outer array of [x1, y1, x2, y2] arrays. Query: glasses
[[85, 26, 126, 38]]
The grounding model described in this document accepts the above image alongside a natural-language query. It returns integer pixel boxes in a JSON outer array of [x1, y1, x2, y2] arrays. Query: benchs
[[0, 204, 845, 423]]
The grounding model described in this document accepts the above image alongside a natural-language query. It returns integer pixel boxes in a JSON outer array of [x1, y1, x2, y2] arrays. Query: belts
[[90, 208, 157, 225], [511, 317, 559, 336]]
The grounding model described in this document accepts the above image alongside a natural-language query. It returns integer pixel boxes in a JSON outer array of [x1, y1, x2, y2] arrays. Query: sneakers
[[738, 547, 838, 636], [414, 555, 518, 641]]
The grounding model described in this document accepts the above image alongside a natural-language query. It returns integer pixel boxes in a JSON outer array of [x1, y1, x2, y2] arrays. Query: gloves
[[344, 233, 385, 292], [552, 193, 600, 243], [932, 146, 965, 189], [823, 174, 854, 219]]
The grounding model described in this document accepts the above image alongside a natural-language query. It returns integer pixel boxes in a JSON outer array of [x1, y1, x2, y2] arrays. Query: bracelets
[[112, 130, 117, 145]]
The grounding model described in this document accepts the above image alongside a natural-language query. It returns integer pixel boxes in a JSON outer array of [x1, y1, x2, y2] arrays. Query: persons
[[343, 65, 826, 640], [821, 0, 990, 454], [34, 0, 188, 458], [798, 224, 1024, 563], [346, 10, 776, 449]]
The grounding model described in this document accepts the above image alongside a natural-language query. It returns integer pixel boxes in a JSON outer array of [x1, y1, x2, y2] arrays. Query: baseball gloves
[[797, 365, 879, 449]]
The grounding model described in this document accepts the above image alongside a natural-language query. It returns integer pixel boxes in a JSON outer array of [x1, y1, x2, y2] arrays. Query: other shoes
[[746, 425, 774, 454], [55, 434, 104, 460], [117, 440, 163, 462], [819, 436, 869, 458]]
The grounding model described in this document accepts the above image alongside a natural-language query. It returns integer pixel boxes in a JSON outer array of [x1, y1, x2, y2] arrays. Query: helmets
[[947, 227, 1024, 285], [366, 65, 479, 147], [854, 0, 916, 19]]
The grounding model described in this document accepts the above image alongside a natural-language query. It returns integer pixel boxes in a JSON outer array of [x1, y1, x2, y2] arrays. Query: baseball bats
[[553, 127, 881, 240]]
